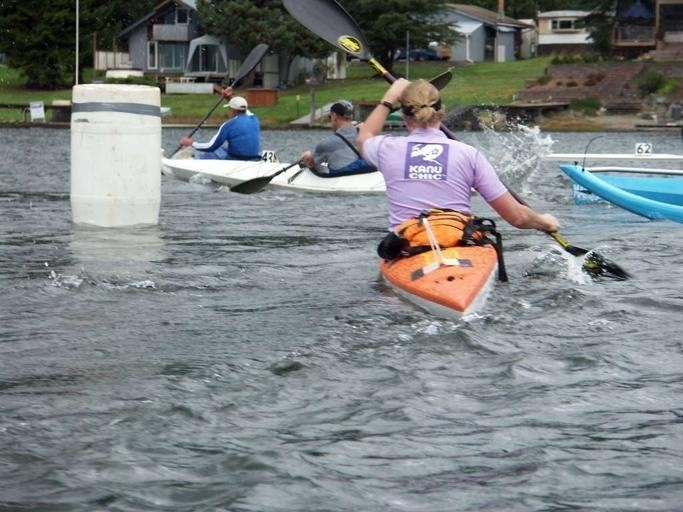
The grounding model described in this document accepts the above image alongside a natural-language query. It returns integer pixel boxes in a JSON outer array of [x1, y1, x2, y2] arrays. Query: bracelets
[[377, 99, 393, 111]]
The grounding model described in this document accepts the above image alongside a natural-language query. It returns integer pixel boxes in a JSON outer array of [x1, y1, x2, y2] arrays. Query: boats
[[559, 160, 683, 222], [160, 153, 545, 195], [377, 210, 501, 323]]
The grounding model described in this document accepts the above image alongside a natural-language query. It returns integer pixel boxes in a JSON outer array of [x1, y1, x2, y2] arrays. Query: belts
[[227, 150, 263, 160]]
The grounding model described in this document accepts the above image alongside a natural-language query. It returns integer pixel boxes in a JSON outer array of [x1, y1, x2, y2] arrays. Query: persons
[[300, 99, 363, 178], [354, 78, 560, 257], [179, 87, 262, 161]]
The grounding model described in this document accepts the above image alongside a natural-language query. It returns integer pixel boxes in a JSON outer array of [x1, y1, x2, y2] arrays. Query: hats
[[326, 100, 354, 115], [223, 96, 249, 111]]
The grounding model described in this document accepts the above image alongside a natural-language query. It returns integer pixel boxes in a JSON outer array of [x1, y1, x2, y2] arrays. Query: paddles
[[284, 0, 633, 282], [167, 43, 268, 158], [229, 72, 452, 194]]
